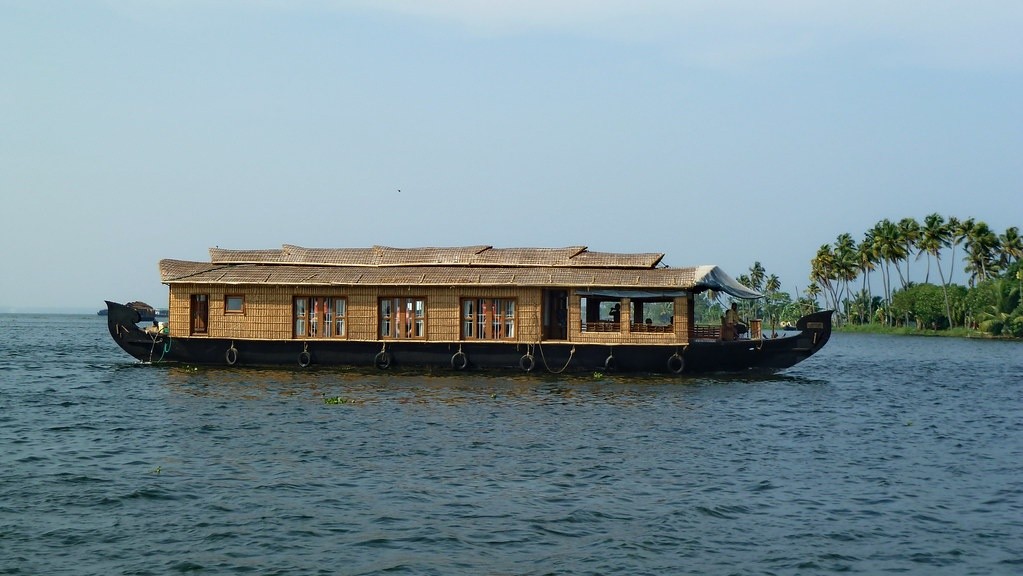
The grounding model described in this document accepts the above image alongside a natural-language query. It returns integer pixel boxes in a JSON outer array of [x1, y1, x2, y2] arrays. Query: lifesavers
[[483, 316, 503, 337], [396, 316, 412, 337], [308, 316, 330, 337]]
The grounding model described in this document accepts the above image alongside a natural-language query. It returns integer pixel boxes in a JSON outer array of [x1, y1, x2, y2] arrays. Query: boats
[[103, 242, 836, 379]]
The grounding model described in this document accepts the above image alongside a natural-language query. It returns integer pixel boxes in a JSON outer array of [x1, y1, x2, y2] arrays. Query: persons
[[645, 319, 655, 332], [153, 321, 168, 330], [728, 303, 749, 340], [609, 304, 620, 323], [670, 316, 673, 324], [724, 309, 730, 325]]
